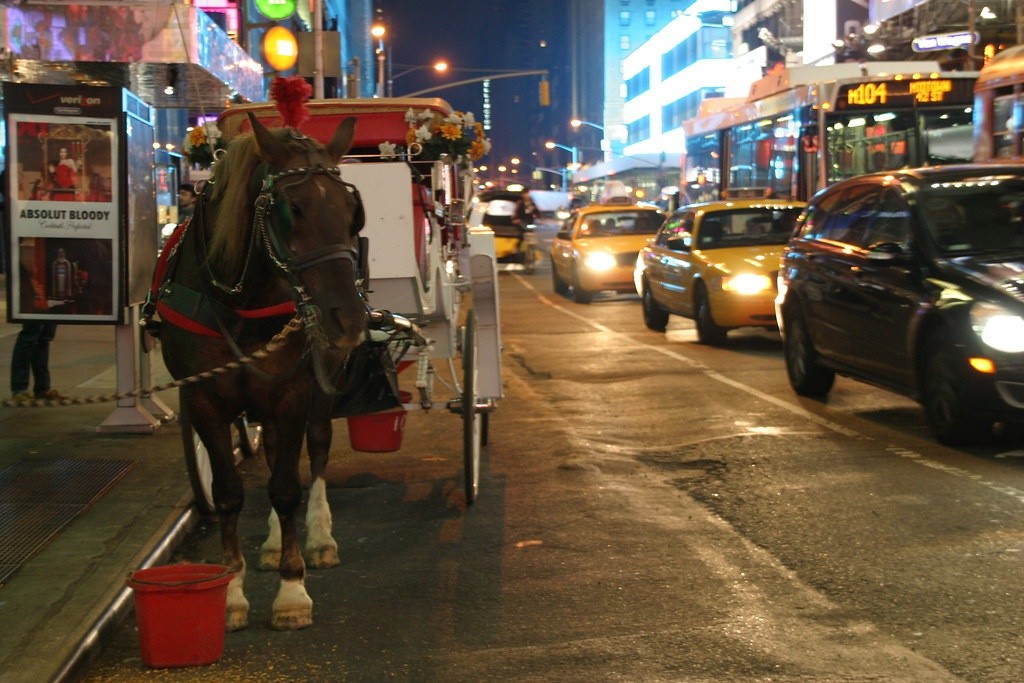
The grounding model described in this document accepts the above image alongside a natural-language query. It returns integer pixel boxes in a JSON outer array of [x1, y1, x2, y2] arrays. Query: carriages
[[139, 75, 505, 633]]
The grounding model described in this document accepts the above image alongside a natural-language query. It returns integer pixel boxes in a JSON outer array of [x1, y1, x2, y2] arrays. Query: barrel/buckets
[[126, 564, 235, 668], [346, 390, 413, 451]]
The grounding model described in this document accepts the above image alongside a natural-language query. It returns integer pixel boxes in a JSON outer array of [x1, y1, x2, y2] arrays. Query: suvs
[[773, 164, 1023, 453]]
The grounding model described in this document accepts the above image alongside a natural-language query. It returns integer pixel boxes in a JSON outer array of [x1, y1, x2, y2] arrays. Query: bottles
[[53, 249, 70, 298]]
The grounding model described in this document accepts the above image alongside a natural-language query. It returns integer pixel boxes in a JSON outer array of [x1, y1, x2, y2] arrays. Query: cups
[[75, 272, 88, 293]]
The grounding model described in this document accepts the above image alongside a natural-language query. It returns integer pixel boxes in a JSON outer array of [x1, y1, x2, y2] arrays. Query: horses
[[157, 110, 368, 630]]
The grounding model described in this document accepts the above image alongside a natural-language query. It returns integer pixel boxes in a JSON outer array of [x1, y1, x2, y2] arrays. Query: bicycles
[[518, 222, 537, 273]]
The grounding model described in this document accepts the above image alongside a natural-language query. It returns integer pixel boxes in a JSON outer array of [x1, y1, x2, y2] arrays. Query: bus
[[677, 59, 983, 207], [971, 43, 1024, 166]]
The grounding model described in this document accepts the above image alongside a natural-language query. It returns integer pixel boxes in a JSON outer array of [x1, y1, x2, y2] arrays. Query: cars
[[478, 189, 528, 262], [551, 203, 668, 304], [634, 197, 809, 348]]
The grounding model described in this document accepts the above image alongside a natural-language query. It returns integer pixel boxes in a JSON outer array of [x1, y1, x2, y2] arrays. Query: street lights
[[545, 141, 578, 172], [370, 25, 385, 98], [388, 47, 448, 99], [533, 165, 567, 194], [569, 119, 609, 188]]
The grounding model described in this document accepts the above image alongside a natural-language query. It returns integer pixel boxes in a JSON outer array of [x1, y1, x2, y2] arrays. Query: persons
[[512, 187, 540, 256], [178, 183, 197, 222], [48, 147, 84, 201], [11, 318, 63, 402]]
